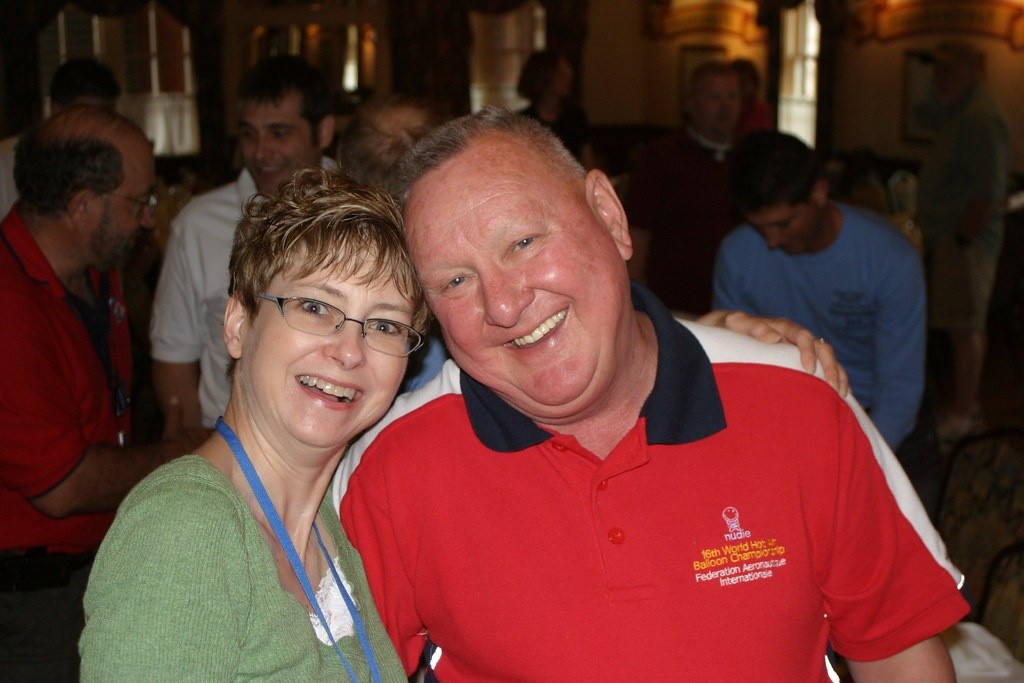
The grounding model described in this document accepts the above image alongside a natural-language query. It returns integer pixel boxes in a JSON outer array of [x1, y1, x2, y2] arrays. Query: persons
[[143, 53, 337, 455], [708, 125, 928, 460], [336, 91, 446, 190], [78, 167, 850, 683], [912, 39, 1011, 443], [0, 101, 217, 682], [513, 52, 600, 174], [2, 52, 123, 222], [338, 109, 975, 681], [726, 59, 775, 152], [622, 61, 747, 319]]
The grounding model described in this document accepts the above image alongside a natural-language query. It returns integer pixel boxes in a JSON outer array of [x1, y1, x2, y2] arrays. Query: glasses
[[106, 192, 158, 215], [256, 291, 428, 359]]
